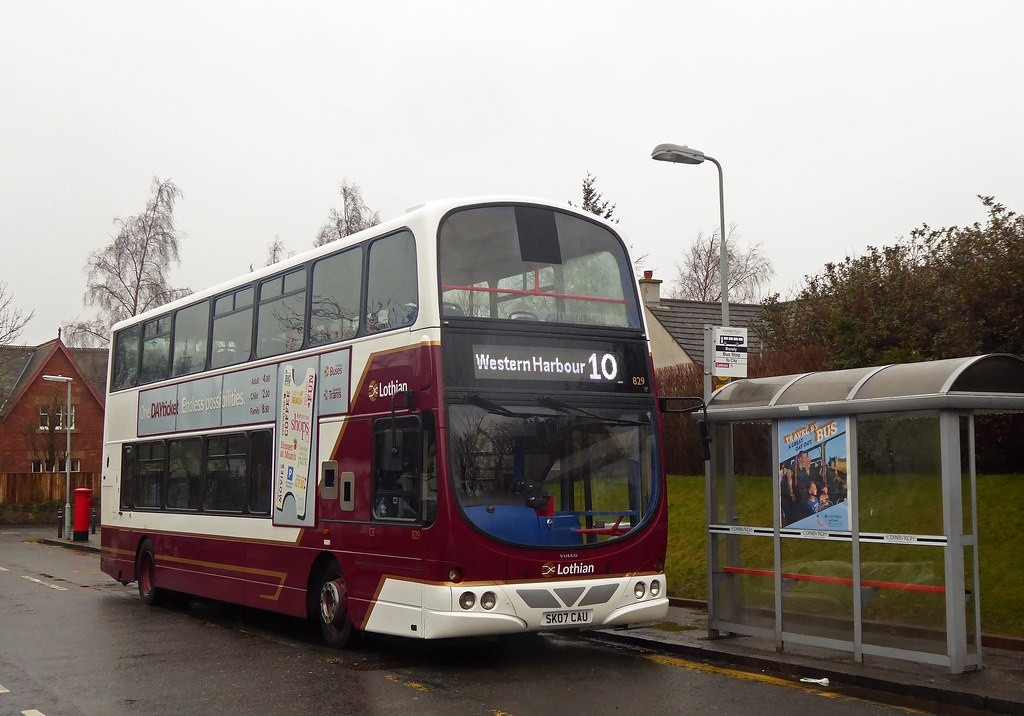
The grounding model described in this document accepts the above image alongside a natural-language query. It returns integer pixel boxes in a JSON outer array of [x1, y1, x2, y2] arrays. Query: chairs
[[131, 467, 247, 515], [111, 300, 547, 387]]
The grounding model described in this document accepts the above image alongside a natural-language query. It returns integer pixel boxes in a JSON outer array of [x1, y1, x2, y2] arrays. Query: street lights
[[651, 142, 735, 565], [42, 374, 73, 539]]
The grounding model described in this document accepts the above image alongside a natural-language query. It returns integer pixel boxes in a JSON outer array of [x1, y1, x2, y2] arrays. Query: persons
[[780, 451, 829, 524]]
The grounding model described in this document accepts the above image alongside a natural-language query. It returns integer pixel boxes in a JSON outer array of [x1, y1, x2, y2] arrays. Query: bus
[[99, 195, 716, 651]]
